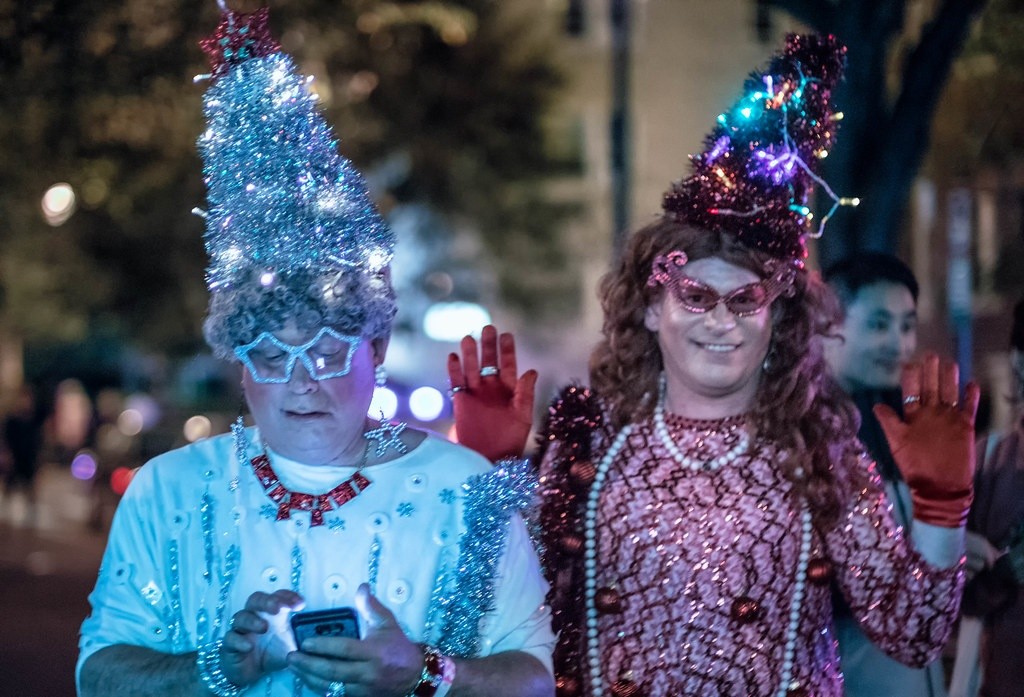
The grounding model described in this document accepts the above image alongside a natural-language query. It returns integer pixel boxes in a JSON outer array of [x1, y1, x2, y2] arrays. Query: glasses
[[234, 325, 371, 383], [650, 250, 803, 317]]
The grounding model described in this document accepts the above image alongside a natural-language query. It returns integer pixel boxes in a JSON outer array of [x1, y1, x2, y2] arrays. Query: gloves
[[447, 324, 538, 464], [872, 351, 980, 528]]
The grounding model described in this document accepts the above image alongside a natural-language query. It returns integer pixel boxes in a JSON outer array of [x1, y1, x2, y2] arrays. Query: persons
[[816, 251, 1024, 697], [0, 387, 40, 530], [75, 0, 558, 697], [447, 31, 980, 697]]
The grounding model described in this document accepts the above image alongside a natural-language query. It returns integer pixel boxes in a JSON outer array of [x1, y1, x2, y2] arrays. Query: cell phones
[[288, 605, 360, 654]]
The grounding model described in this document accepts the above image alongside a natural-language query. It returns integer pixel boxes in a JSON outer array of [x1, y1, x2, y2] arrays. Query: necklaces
[[249, 415, 372, 527], [655, 368, 766, 470], [586, 372, 812, 697]]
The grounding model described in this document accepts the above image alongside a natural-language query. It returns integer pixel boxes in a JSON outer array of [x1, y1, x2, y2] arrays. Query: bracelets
[[197, 638, 250, 697], [405, 642, 456, 697]]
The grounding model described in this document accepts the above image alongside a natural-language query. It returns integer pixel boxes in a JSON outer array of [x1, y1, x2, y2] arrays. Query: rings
[[941, 400, 960, 408], [448, 385, 467, 397], [229, 611, 248, 635], [901, 396, 921, 403], [325, 682, 345, 697], [479, 366, 499, 376]]
